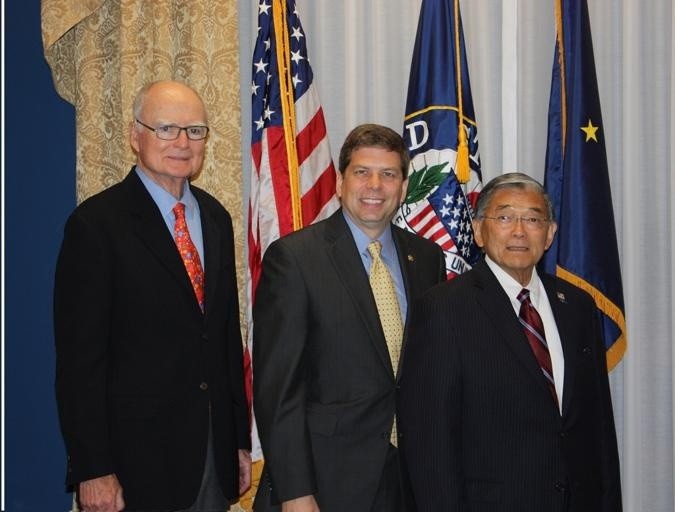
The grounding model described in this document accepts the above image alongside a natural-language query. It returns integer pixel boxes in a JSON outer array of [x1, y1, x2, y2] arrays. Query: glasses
[[478, 211, 551, 232], [136, 119, 210, 141]]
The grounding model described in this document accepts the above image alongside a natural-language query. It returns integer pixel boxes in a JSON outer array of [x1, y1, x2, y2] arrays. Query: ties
[[517, 288, 559, 421], [368, 241, 405, 449], [173, 202, 205, 314]]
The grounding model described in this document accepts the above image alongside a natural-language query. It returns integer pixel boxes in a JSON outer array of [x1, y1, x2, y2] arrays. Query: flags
[[240, 0, 346, 446], [539, 0, 629, 374], [396, 0, 486, 282]]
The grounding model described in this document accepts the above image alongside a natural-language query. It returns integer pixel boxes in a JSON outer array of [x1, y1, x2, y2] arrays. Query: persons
[[252, 124, 447, 511], [50, 79, 253, 511], [402, 171, 624, 511]]
